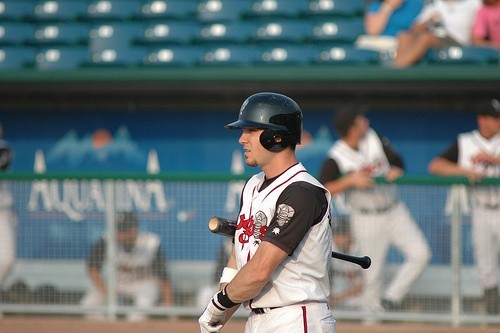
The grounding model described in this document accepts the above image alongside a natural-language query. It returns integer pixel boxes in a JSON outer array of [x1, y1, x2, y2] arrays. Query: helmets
[[222, 92, 302, 152]]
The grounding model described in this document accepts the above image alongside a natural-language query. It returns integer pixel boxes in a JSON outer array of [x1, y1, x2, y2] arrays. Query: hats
[[0, 140, 11, 150], [335, 106, 367, 129], [117, 212, 136, 229], [476, 98, 500, 117]]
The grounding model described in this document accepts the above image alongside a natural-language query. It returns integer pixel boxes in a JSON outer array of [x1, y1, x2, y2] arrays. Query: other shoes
[[379, 299, 396, 312]]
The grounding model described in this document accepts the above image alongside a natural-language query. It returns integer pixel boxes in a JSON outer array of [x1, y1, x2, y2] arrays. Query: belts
[[253, 306, 276, 315]]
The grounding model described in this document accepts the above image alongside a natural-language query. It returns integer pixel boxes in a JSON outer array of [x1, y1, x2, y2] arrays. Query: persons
[[80, 213, 180, 322], [428, 96, 500, 317], [0, 145, 15, 288], [318, 105, 432, 325], [197, 92, 337, 333], [362, 0, 500, 68]]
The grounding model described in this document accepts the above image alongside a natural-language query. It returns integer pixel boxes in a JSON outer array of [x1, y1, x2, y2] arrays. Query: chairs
[[0, 0, 500, 67]]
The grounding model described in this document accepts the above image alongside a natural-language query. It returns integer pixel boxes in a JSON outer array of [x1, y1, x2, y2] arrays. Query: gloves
[[198, 288, 237, 333]]
